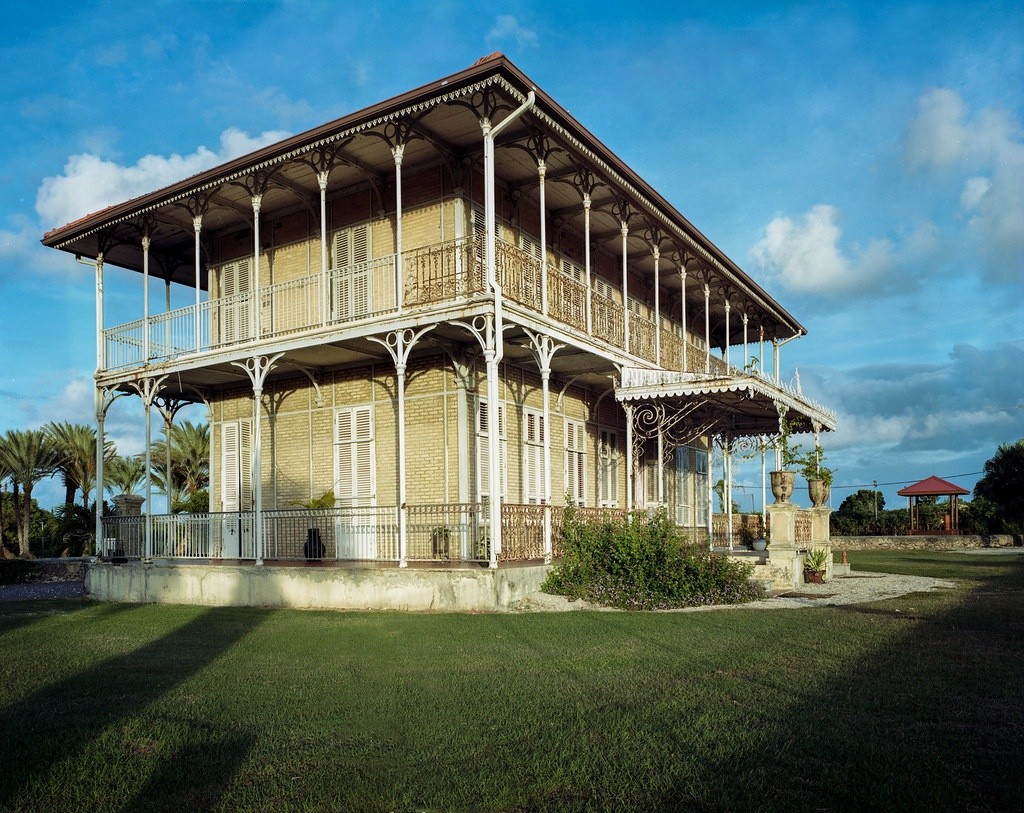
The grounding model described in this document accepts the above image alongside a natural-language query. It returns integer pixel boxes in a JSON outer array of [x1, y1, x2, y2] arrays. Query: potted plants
[[753, 513, 766, 550], [428, 526, 451, 552], [743, 405, 806, 504], [473, 536, 491, 567], [287, 489, 336, 561], [801, 445, 838, 507], [803, 547, 830, 583]]
